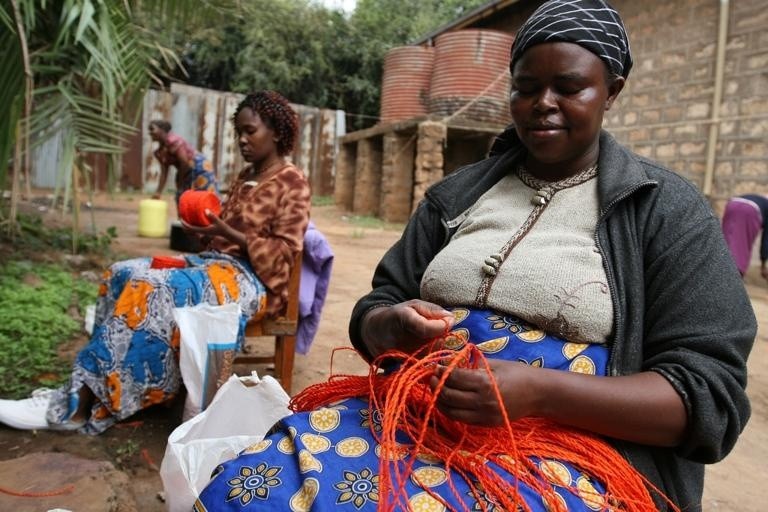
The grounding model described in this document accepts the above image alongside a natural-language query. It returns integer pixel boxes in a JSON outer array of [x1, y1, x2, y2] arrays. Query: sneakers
[[0, 387, 86, 432]]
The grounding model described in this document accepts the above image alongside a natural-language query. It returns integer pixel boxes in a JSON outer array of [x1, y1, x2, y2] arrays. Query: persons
[[722, 193, 768, 282], [148, 118, 220, 217], [0, 88, 311, 439], [186, 1, 758, 512]]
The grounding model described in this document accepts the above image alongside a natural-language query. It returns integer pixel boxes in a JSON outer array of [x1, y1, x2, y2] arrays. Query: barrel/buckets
[[169, 223, 200, 253], [138, 199, 168, 238]]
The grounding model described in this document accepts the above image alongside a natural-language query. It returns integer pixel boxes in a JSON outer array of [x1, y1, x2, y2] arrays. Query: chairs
[[241, 251, 303, 395]]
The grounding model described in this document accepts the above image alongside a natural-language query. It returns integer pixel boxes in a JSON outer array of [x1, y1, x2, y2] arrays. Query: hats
[[506, 1, 634, 78]]
